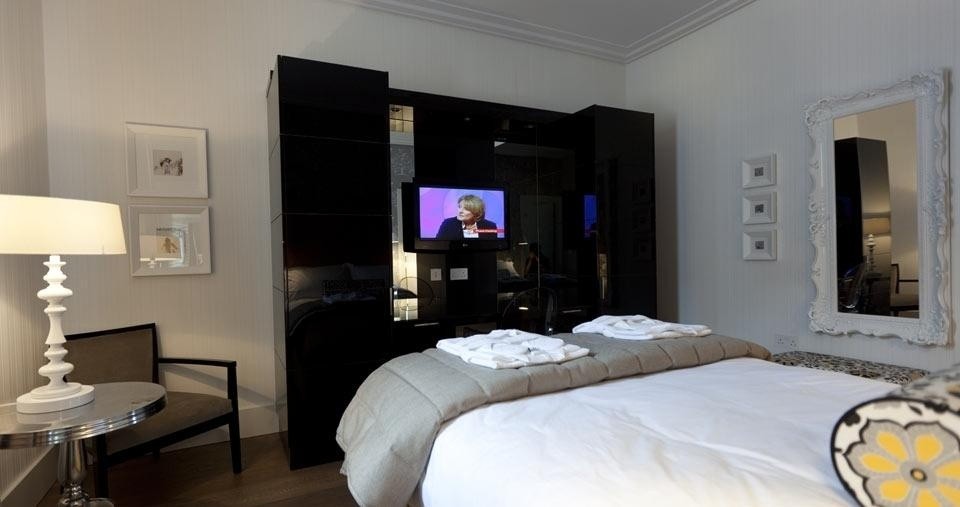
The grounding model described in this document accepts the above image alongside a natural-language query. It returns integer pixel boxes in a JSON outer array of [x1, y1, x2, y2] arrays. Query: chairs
[[889, 263, 919, 316], [62, 323, 242, 497]]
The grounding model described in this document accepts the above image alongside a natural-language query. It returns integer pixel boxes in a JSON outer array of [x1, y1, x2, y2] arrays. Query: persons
[[436, 195, 497, 238]]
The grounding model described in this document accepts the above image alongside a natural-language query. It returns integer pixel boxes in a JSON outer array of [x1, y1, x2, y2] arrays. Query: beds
[[336, 323, 960, 507]]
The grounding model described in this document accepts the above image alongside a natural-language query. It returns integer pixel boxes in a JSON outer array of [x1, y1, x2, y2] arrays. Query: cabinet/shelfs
[[266, 55, 658, 470]]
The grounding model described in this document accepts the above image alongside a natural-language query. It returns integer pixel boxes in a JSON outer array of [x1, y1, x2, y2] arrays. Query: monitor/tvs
[[401, 181, 512, 253]]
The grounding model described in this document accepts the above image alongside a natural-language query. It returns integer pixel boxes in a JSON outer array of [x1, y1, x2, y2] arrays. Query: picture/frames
[[740, 153, 777, 262], [123, 121, 213, 278]]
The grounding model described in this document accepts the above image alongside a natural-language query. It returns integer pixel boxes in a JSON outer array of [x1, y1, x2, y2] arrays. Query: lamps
[[0, 193, 128, 415]]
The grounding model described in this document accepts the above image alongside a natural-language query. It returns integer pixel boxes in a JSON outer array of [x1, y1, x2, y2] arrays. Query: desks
[[1, 381, 166, 507]]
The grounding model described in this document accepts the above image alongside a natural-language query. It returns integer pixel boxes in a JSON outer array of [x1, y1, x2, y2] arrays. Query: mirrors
[[798, 67, 955, 348]]
